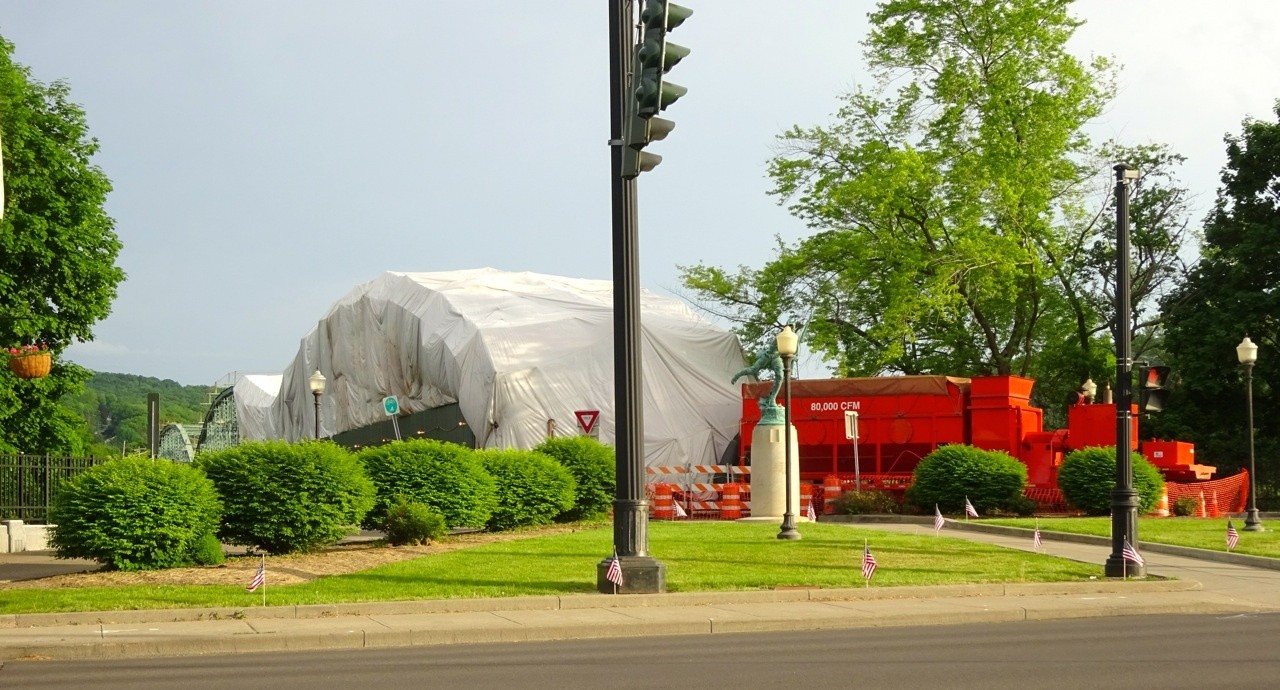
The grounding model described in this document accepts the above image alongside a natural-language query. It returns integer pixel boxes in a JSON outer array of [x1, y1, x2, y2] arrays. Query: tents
[[281, 268, 762, 503]]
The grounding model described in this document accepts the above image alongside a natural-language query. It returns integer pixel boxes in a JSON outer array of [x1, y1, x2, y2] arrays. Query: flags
[[246, 558, 265, 593], [1034, 529, 1044, 550], [672, 499, 687, 517], [1227, 521, 1239, 549], [606, 551, 623, 587], [862, 548, 877, 580], [966, 499, 979, 518], [935, 507, 945, 532], [1123, 540, 1145, 568], [807, 501, 816, 520]]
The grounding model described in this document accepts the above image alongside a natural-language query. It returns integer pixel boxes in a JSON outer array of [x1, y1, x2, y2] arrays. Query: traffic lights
[[620, 85, 663, 181], [634, 1, 695, 121], [623, 42, 675, 153]]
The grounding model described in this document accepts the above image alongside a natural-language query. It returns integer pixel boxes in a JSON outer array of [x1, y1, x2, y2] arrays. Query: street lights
[[1236, 336, 1264, 532], [308, 370, 327, 442], [776, 326, 803, 540], [1103, 164, 1146, 580]]
